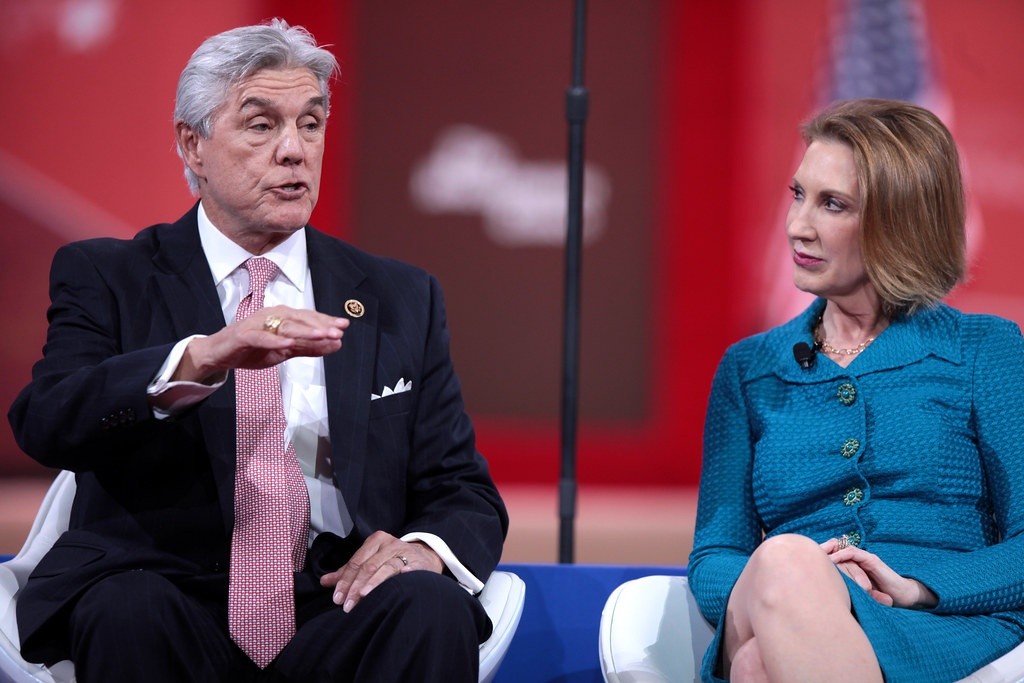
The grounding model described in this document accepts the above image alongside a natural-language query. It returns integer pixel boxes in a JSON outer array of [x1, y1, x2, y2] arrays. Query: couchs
[[0, 554, 1024, 682]]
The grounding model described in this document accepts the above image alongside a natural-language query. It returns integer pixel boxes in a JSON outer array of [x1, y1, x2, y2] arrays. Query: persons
[[687, 99, 1024, 683], [7, 19, 509, 683]]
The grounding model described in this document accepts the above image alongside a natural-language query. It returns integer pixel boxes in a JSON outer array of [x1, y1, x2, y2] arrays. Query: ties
[[228, 256, 311, 671]]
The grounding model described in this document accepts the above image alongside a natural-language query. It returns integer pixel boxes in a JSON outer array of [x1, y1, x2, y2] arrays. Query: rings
[[838, 537, 850, 550], [396, 556, 408, 566], [263, 314, 285, 335]]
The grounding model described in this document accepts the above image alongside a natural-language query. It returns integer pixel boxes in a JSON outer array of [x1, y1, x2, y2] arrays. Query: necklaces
[[814, 316, 887, 355]]
